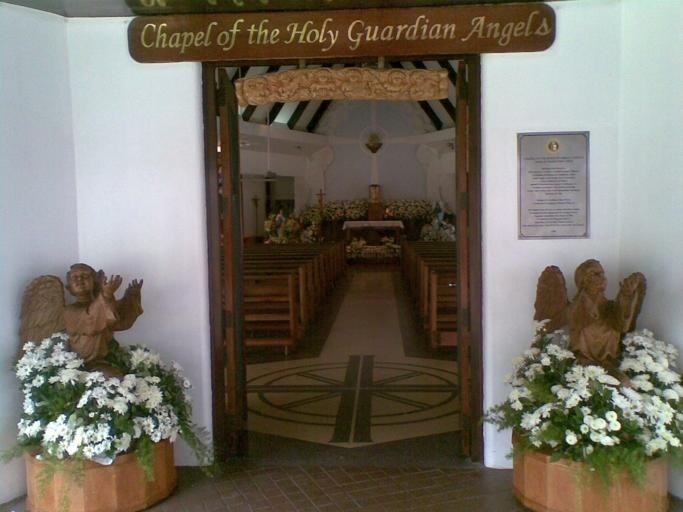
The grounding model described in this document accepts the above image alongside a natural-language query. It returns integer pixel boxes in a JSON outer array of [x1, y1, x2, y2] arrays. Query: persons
[[274, 204, 286, 236], [561, 256, 649, 391], [58, 260, 144, 380]]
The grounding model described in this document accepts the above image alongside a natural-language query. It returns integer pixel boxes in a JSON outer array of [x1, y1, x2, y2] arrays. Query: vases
[[511, 426, 670, 512], [22, 439, 177, 511]]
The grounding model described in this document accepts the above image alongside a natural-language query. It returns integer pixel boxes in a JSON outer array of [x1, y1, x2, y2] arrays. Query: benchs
[[400, 239, 457, 349], [217, 238, 347, 359]]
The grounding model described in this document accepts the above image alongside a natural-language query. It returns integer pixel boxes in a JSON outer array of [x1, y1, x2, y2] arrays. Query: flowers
[[457, 318, 682, 512], [0, 331, 236, 511]]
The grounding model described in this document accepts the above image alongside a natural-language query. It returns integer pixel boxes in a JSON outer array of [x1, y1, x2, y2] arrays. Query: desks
[[342, 220, 402, 242]]
